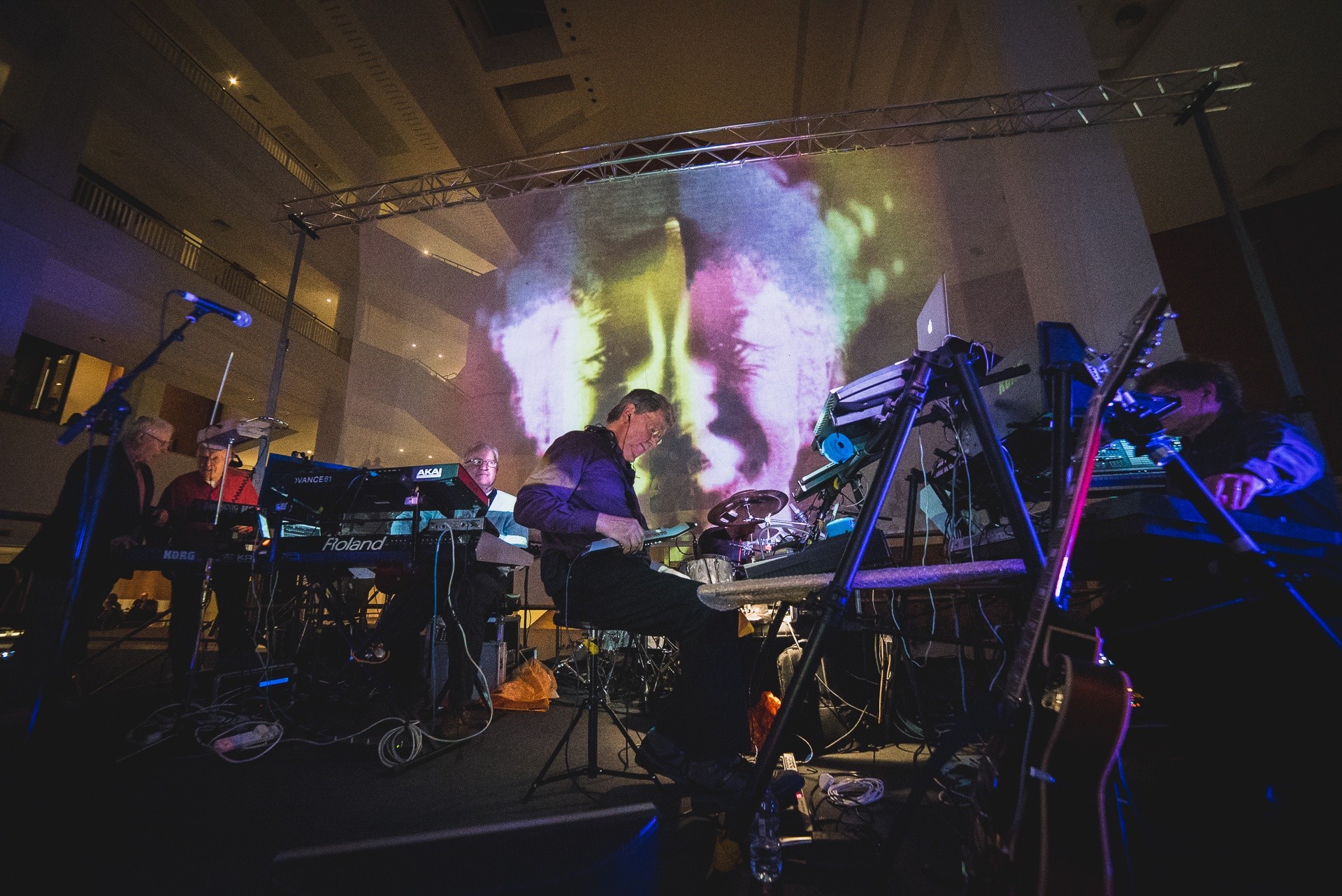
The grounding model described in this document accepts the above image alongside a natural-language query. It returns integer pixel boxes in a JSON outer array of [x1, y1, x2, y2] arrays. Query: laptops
[[917, 271, 951, 351]]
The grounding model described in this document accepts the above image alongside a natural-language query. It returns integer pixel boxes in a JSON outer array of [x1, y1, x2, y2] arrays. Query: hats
[[197, 426, 228, 450]]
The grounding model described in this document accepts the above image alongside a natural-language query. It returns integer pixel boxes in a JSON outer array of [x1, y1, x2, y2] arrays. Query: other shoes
[[66, 665, 95, 700], [634, 728, 737, 795], [215, 667, 255, 699], [165, 673, 195, 703]]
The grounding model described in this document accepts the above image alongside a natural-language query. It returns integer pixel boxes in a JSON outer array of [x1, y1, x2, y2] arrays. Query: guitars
[[957, 292, 1177, 896]]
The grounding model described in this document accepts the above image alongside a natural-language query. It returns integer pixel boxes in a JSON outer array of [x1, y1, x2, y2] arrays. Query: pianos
[[234, 462, 534, 737]]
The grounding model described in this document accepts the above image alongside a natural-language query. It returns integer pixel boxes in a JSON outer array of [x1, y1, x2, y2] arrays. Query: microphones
[[175, 288, 253, 327]]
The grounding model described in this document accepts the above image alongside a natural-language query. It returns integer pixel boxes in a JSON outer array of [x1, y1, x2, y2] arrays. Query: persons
[[0, 415, 174, 764], [290, 450, 315, 462], [514, 390, 805, 813], [1132, 355, 1342, 583], [347, 440, 529, 715], [128, 593, 154, 622], [359, 457, 386, 470], [98, 593, 122, 630], [155, 440, 262, 687], [22, 393, 112, 433], [492, 163, 841, 561]]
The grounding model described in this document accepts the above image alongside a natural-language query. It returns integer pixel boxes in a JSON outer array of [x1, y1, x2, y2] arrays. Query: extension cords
[[214, 726, 280, 753]]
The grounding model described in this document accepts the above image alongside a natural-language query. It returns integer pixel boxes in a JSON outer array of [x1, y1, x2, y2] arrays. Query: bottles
[[750, 785, 782, 882]]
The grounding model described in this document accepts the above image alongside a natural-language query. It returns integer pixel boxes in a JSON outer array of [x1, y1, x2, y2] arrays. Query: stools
[[521, 613, 679, 807]]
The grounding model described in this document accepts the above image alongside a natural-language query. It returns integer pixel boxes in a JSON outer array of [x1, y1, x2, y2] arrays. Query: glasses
[[143, 432, 174, 449], [465, 458, 497, 469], [644, 415, 663, 446]]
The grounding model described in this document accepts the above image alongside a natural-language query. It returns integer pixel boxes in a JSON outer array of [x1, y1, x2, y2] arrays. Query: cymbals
[[707, 489, 788, 526]]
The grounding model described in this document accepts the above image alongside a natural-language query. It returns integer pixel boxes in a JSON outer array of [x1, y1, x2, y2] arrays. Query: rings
[[1232, 486, 1243, 492]]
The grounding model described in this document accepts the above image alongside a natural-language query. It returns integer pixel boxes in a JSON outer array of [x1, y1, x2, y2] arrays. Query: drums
[[676, 554, 735, 583], [586, 630, 666, 669]]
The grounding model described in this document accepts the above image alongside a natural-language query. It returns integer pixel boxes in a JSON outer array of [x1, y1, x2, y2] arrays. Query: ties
[[136, 468, 146, 514]]
